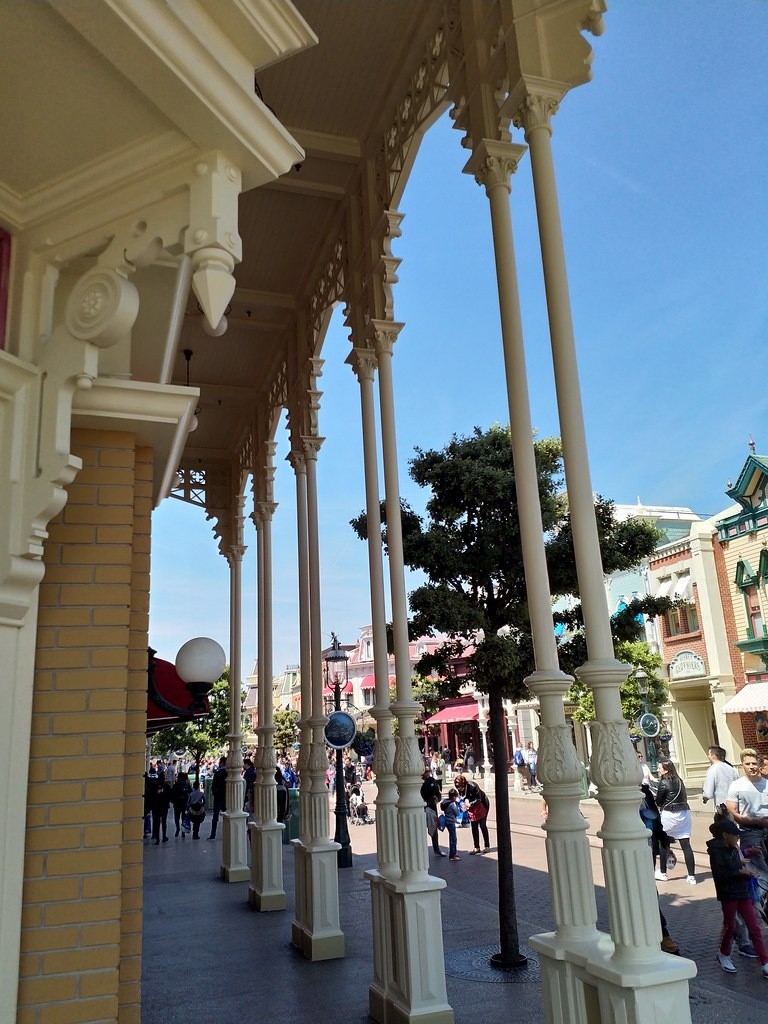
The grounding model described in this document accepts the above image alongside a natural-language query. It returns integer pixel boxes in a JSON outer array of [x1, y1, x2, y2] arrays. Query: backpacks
[[514, 749, 524, 766], [439, 815, 445, 832]]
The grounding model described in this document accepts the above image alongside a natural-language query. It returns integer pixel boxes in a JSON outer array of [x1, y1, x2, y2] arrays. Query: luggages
[[143, 814, 152, 839]]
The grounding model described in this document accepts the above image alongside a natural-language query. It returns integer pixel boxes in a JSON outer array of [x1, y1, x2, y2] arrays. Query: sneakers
[[762, 964, 768, 978], [654, 870, 668, 880], [207, 836, 215, 841], [687, 875, 696, 884], [738, 944, 759, 957], [661, 936, 679, 952], [449, 854, 462, 860], [714, 948, 737, 973]]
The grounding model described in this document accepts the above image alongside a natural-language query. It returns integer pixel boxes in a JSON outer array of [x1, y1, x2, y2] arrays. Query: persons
[[242, 749, 256, 843], [143, 756, 228, 845], [703, 745, 739, 824], [422, 744, 451, 792], [421, 765, 490, 860], [706, 803, 768, 979], [514, 742, 544, 793], [325, 747, 336, 797], [343, 748, 373, 820], [726, 748, 768, 957], [657, 890, 679, 952], [636, 751, 696, 884], [275, 752, 300, 820], [453, 744, 474, 774]]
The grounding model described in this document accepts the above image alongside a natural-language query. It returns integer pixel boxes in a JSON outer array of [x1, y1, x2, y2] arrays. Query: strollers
[[757, 834, 768, 926], [346, 784, 374, 826]]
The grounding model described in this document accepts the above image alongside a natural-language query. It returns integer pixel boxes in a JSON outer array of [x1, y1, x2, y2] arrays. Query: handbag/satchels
[[639, 799, 657, 830], [467, 803, 486, 822], [436, 767, 442, 775], [181, 817, 191, 833], [747, 877, 761, 905]]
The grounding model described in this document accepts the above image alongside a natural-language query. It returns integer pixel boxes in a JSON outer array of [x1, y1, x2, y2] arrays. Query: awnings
[[360, 675, 396, 689], [146, 656, 210, 739], [323, 687, 335, 697], [342, 682, 353, 695], [721, 683, 768, 713], [425, 704, 490, 724]]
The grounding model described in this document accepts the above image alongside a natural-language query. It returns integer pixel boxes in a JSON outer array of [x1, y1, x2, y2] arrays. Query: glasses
[[638, 755, 643, 758]]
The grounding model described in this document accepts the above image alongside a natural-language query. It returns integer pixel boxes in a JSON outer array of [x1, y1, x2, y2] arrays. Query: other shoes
[[470, 848, 480, 854], [483, 847, 490, 853], [193, 836, 200, 839], [434, 852, 446, 856], [175, 828, 180, 837]]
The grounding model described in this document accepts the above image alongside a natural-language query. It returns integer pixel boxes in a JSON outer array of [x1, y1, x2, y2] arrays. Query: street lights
[[242, 735, 247, 760], [324, 635, 352, 868], [294, 725, 300, 762], [633, 662, 661, 789]]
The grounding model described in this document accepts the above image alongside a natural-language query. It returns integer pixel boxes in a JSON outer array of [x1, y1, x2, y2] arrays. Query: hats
[[718, 820, 742, 834]]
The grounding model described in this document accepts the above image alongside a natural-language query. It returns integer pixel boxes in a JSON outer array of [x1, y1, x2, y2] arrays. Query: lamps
[[147, 637, 226, 718]]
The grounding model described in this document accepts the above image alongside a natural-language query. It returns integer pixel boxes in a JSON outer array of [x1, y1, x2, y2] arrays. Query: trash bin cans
[[440, 759, 446, 785], [282, 788, 300, 845], [204, 779, 215, 813]]
